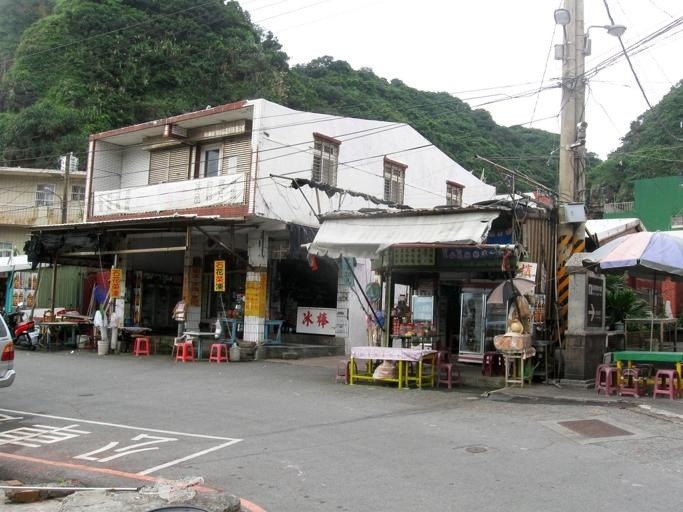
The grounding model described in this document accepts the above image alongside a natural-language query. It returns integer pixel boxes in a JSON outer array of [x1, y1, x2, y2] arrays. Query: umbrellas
[[484, 274, 536, 323], [581, 229, 683, 351]]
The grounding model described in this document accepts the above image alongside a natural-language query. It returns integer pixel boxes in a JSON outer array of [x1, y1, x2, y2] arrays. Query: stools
[[595, 364, 683, 400], [209, 344, 229, 363], [175, 343, 195, 364], [482, 352, 504, 377], [504, 348, 533, 387], [133, 338, 150, 357], [336, 360, 357, 386]]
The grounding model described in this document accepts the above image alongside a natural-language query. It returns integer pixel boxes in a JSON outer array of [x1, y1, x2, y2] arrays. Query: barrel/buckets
[[113, 341, 121, 353], [97, 341, 109, 356], [28, 332, 40, 346], [230, 342, 241, 361], [78, 335, 88, 348]]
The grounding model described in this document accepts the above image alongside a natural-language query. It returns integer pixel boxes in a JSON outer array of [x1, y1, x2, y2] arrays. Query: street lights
[[554, 8, 626, 349]]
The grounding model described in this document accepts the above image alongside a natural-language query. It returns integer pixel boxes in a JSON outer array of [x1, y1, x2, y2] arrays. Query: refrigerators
[[296, 306, 337, 336], [460, 282, 509, 365]]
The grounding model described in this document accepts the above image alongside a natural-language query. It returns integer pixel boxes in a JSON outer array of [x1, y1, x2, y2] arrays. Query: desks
[[349, 347, 435, 390], [624, 318, 679, 351], [40, 321, 90, 349]]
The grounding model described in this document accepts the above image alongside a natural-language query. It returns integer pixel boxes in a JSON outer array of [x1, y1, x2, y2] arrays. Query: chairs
[[436, 351, 462, 390]]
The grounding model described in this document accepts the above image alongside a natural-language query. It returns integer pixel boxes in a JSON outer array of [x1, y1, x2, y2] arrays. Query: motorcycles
[[0, 302, 42, 351]]
